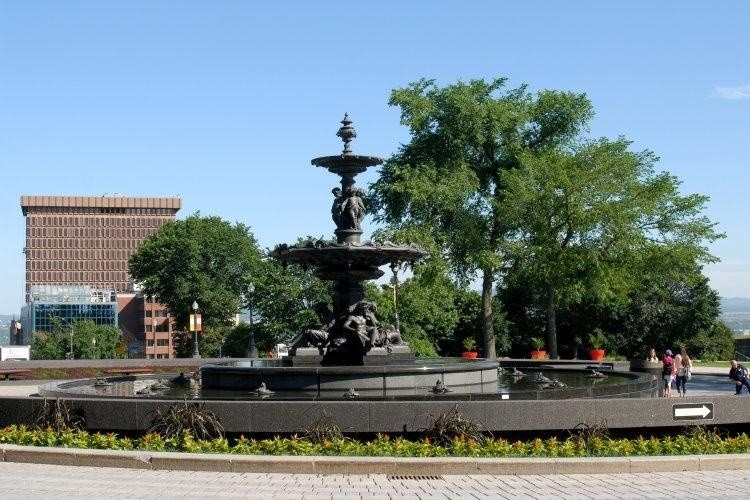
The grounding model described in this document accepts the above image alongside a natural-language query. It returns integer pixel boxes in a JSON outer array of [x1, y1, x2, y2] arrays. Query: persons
[[330, 187, 367, 230], [267, 350, 274, 358], [289, 297, 411, 355], [728, 360, 750, 395], [645, 347, 659, 363], [662, 348, 692, 397]]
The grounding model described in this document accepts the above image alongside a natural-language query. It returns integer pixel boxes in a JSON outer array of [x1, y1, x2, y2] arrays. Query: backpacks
[[663, 357, 673, 375]]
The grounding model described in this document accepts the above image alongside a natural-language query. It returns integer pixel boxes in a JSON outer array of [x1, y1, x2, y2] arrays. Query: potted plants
[[462, 337, 479, 359], [587, 327, 608, 360], [530, 336, 546, 359]]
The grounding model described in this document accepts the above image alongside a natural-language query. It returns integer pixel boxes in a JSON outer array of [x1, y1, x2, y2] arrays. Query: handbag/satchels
[[685, 365, 692, 382]]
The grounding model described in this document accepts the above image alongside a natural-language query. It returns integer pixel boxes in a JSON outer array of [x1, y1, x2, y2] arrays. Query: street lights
[[192, 300, 199, 357], [70, 329, 74, 354], [390, 261, 400, 310], [247, 282, 255, 356]]
